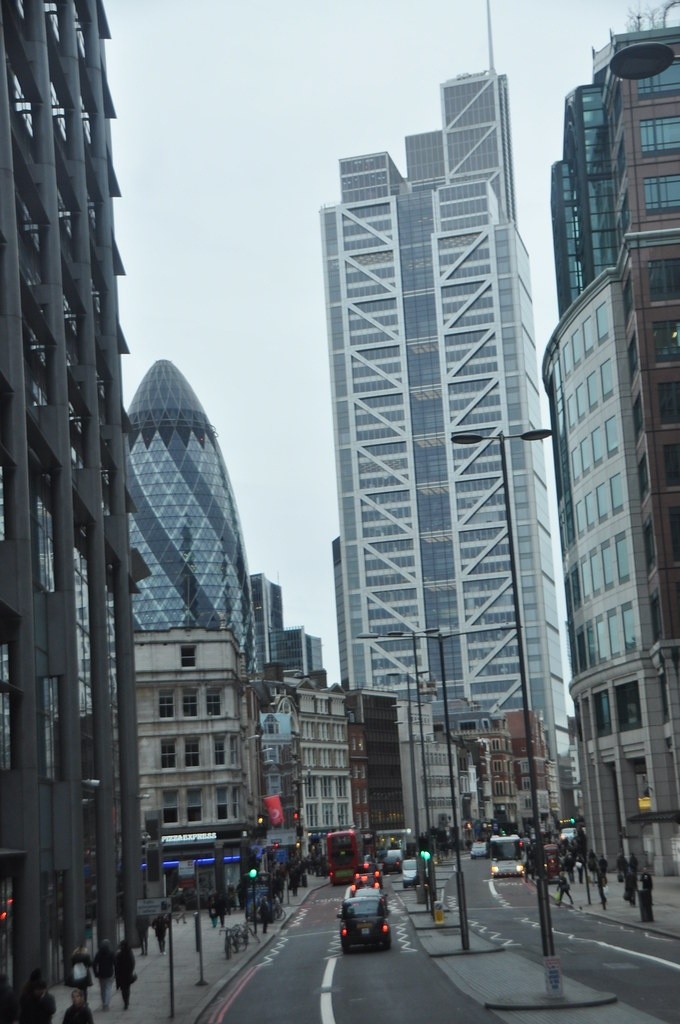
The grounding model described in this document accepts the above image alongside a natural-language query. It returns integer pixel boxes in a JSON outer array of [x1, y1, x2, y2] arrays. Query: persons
[[616, 853, 628, 878], [208, 879, 244, 927], [176, 895, 187, 923], [624, 872, 637, 906], [555, 872, 574, 906], [136, 915, 149, 956], [0, 968, 56, 1024], [629, 852, 638, 875], [524, 814, 608, 883], [71, 943, 94, 1003], [640, 868, 654, 905], [151, 914, 169, 955], [93, 938, 117, 1012], [62, 989, 95, 1024], [114, 940, 137, 1010], [271, 854, 328, 904], [260, 895, 268, 933]]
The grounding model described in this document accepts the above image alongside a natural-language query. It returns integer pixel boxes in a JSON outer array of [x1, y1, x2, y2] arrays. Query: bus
[[490, 834, 522, 878], [326, 831, 359, 886]]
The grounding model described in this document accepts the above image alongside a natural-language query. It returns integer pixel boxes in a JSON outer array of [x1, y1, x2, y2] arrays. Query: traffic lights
[[258, 814, 268, 829], [294, 812, 299, 820], [248, 862, 258, 879], [419, 833, 430, 861], [570, 817, 575, 825]]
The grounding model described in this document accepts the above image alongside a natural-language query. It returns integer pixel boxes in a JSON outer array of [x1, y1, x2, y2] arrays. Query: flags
[[264, 794, 284, 826]]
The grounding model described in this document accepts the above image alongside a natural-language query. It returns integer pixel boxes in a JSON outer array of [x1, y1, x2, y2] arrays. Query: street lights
[[356, 623, 519, 950], [387, 629, 440, 921], [452, 430, 565, 1002]]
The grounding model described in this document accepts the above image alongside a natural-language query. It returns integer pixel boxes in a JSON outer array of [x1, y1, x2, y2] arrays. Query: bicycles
[[274, 902, 286, 922], [219, 923, 261, 953]]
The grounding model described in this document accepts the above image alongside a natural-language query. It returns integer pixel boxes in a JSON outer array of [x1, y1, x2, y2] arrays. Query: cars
[[337, 864, 391, 955], [364, 850, 429, 888], [470, 842, 489, 860], [520, 833, 536, 850]]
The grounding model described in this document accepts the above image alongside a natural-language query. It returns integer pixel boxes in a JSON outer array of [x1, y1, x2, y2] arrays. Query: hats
[[30, 968, 42, 980]]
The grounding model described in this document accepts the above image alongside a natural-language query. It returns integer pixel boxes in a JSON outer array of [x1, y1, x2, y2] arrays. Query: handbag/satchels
[[618, 872, 623, 882], [624, 890, 632, 900], [211, 909, 216, 914], [555, 891, 561, 901], [128, 973, 136, 983], [562, 884, 570, 891], [74, 962, 87, 980], [576, 861, 581, 869]]
